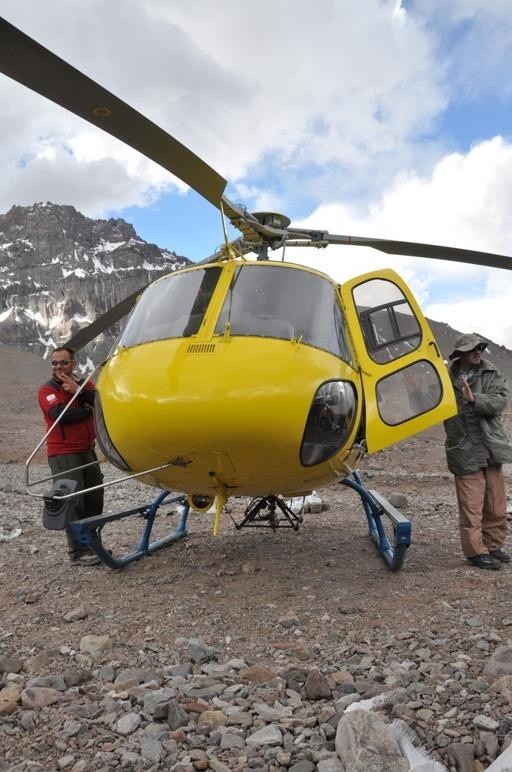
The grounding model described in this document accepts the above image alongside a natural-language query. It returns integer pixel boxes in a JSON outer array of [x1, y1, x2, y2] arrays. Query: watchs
[[75, 387, 82, 395]]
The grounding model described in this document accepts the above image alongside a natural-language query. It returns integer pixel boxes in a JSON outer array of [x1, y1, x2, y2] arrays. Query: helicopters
[[0, 16, 512, 571]]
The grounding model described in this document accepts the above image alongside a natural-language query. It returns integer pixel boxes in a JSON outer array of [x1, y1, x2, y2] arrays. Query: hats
[[448, 334, 489, 360]]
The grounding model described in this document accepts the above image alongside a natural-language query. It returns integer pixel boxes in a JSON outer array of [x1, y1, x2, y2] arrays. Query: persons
[[400, 333, 512, 570], [38, 347, 113, 566]]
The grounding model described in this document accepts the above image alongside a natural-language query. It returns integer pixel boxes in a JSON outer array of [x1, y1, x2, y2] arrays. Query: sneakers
[[69, 549, 112, 565], [466, 550, 510, 569]]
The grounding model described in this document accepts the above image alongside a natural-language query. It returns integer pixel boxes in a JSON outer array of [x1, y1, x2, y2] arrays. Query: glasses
[[51, 359, 71, 366]]
